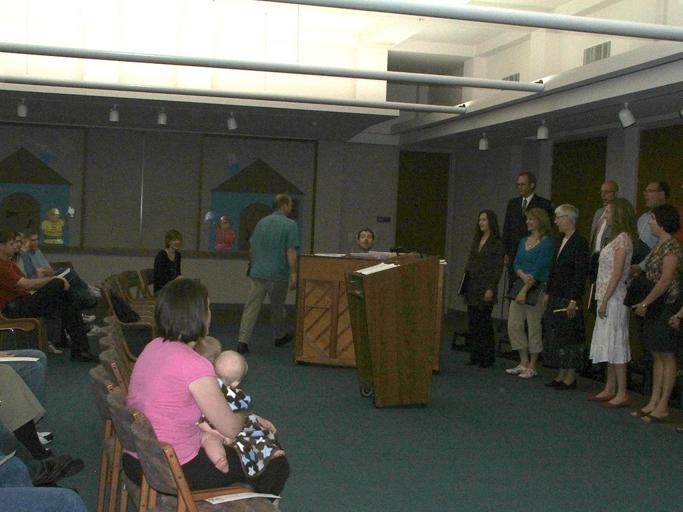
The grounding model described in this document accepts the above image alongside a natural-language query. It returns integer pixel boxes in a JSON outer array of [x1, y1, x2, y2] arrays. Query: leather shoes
[[602, 398, 630, 408], [587, 394, 616, 401]]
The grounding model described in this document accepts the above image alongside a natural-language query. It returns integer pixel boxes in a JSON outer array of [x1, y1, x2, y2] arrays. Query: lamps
[[16, 97, 238, 131], [478, 102, 637, 152]]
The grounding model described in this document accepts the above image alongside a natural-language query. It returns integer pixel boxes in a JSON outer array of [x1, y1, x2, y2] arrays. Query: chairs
[[0, 310, 49, 359], [88, 268, 250, 511]]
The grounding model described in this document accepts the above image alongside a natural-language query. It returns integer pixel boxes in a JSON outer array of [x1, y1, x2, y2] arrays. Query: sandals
[[630, 409, 652, 417], [640, 412, 672, 423]]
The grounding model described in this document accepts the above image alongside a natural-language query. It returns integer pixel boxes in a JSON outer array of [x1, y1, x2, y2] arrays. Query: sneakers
[[86, 325, 106, 337], [480, 359, 496, 368], [32, 453, 85, 487], [82, 312, 96, 324], [70, 350, 98, 363], [238, 342, 249, 354], [275, 333, 293, 348], [518, 368, 538, 379], [48, 342, 64, 355], [36, 431, 53, 446], [464, 358, 480, 365], [504, 364, 527, 375]]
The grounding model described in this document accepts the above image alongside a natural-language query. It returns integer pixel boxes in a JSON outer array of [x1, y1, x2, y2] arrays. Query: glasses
[[643, 188, 657, 194], [599, 190, 615, 194]]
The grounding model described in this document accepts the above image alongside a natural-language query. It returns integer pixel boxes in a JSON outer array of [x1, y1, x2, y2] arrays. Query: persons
[[0, 419, 84, 486], [0, 348, 47, 403], [153, 230, 182, 295], [197, 351, 285, 473], [214, 215, 236, 252], [0, 365, 53, 458], [357, 228, 375, 252], [458, 172, 683, 423], [121, 277, 290, 503], [0, 227, 101, 361], [238, 193, 299, 355], [0, 453, 89, 511], [194, 337, 222, 365], [39, 208, 65, 246]]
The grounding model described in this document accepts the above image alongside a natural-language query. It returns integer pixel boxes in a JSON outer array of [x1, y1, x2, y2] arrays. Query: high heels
[[555, 378, 577, 390], [544, 379, 561, 386]]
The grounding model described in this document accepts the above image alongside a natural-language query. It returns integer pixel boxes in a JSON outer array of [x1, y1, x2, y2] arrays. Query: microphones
[[389, 246, 419, 253]]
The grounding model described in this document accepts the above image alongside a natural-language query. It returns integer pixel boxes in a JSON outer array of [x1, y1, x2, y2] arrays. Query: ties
[[522, 197, 528, 217], [595, 215, 607, 237]]
